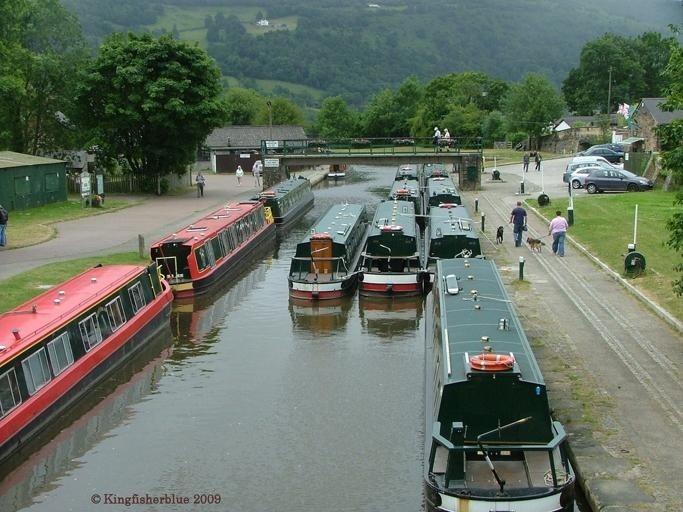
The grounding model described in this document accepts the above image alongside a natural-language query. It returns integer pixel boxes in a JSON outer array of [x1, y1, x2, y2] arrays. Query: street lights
[[266, 100, 272, 140]]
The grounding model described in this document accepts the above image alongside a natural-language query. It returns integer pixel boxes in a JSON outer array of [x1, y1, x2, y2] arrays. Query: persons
[[252, 164, 261, 187], [441, 128, 451, 151], [0, 205, 8, 246], [509, 202, 527, 246], [433, 127, 442, 152], [535, 152, 542, 171], [548, 211, 568, 257], [196, 172, 205, 197], [523, 152, 530, 172], [236, 166, 244, 186]]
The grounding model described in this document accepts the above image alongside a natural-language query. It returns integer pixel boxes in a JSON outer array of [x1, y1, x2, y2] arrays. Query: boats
[[387, 179, 425, 227], [421, 201, 485, 287], [149, 200, 275, 299], [421, 163, 449, 192], [395, 163, 420, 186], [421, 260, 577, 512], [0, 327, 176, 511], [326, 174, 350, 189], [416, 176, 461, 228], [0, 257, 173, 449], [357, 199, 424, 295], [328, 161, 350, 180], [270, 200, 314, 246], [288, 290, 357, 337], [248, 176, 312, 222], [168, 228, 277, 344], [356, 288, 425, 340], [289, 202, 371, 299]]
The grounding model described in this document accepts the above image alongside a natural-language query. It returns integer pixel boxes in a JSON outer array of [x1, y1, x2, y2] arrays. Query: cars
[[584, 169, 653, 194], [572, 155, 617, 170], [569, 168, 605, 187], [576, 148, 624, 166], [583, 143, 626, 155], [562, 162, 602, 184]]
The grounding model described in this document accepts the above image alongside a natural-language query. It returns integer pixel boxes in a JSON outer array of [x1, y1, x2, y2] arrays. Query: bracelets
[[525, 225, 527, 226]]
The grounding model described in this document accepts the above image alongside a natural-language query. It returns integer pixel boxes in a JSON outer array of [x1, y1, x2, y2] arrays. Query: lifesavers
[[397, 188, 409, 193], [439, 203, 456, 208], [469, 354, 514, 372], [312, 233, 330, 240], [379, 225, 403, 231]]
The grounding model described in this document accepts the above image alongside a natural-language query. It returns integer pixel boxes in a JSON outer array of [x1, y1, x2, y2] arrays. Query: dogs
[[495, 225, 504, 244], [525, 237, 547, 253]]
